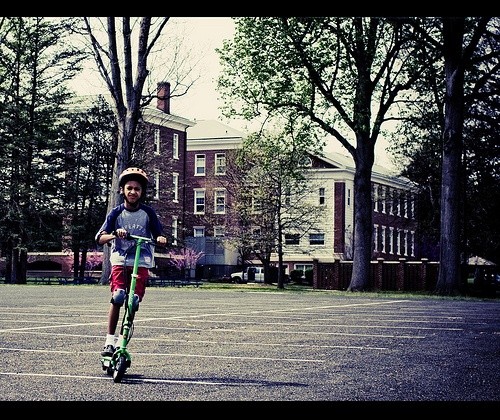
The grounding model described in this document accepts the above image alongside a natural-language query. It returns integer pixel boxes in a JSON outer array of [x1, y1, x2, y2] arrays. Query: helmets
[[119, 167, 150, 183]]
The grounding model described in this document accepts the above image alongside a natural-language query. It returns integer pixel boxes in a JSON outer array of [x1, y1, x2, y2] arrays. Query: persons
[[91, 168, 170, 355]]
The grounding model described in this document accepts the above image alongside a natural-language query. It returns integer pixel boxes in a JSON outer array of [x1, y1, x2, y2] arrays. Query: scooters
[[101, 231, 174, 381]]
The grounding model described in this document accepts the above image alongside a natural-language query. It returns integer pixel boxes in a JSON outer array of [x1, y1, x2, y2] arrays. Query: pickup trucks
[[231, 267, 265, 285]]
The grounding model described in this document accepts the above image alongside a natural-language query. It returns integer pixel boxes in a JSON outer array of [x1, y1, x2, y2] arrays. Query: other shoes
[[102, 345, 115, 356]]
